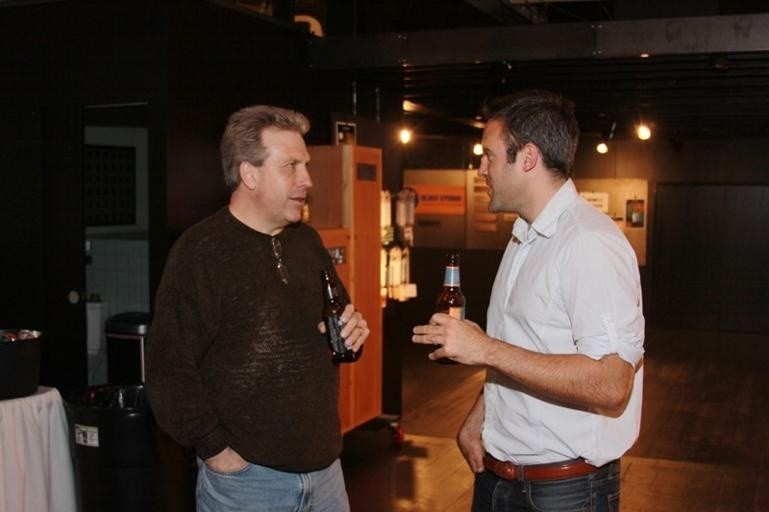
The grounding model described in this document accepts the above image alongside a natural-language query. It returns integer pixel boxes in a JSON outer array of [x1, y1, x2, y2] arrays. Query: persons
[[405, 90, 649, 512], [141, 103, 373, 512]]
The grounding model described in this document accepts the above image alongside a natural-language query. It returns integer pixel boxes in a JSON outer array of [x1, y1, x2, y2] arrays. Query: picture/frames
[[81, 143, 137, 226]]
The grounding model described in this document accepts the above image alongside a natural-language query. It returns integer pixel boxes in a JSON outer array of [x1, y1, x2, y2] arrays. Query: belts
[[481, 453, 595, 484]]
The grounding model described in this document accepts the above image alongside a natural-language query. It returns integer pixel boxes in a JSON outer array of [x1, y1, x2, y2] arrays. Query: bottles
[[431, 254, 467, 365], [319, 268, 361, 364]]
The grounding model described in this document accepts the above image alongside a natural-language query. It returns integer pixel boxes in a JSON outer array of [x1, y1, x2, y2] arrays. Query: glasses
[[272, 236, 289, 286]]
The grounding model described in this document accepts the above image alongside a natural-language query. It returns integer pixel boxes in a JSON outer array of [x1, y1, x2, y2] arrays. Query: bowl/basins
[[70, 383, 153, 471], [1, 325, 48, 400]]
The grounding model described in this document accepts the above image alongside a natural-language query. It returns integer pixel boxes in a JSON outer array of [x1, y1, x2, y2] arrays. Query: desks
[[0, 385, 77, 512]]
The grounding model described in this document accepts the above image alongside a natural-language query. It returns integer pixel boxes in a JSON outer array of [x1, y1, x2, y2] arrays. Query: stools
[[105, 312, 152, 382]]
